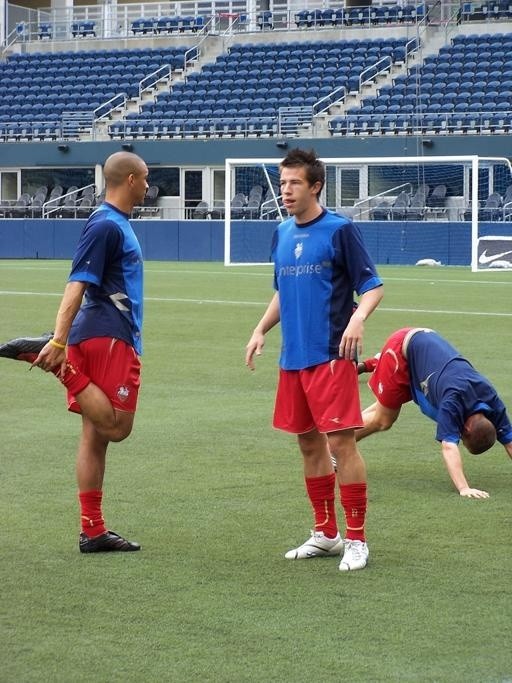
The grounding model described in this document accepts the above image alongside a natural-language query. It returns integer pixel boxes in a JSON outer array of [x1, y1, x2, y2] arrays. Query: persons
[[244, 148, 384, 573], [0, 152, 150, 553], [331, 327, 512, 498]]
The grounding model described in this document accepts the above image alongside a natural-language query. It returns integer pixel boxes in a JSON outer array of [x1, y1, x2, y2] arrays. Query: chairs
[[0, 177, 160, 217], [194, 183, 288, 218], [0, 1, 512, 140], [369, 183, 511, 220]]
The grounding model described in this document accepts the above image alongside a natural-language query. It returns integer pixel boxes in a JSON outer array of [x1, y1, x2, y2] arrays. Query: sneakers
[[0, 333, 53, 363], [285, 530, 369, 570], [80, 530, 140, 552]]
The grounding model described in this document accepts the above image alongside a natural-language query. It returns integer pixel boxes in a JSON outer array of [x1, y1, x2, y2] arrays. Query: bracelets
[[49, 339, 66, 350]]
